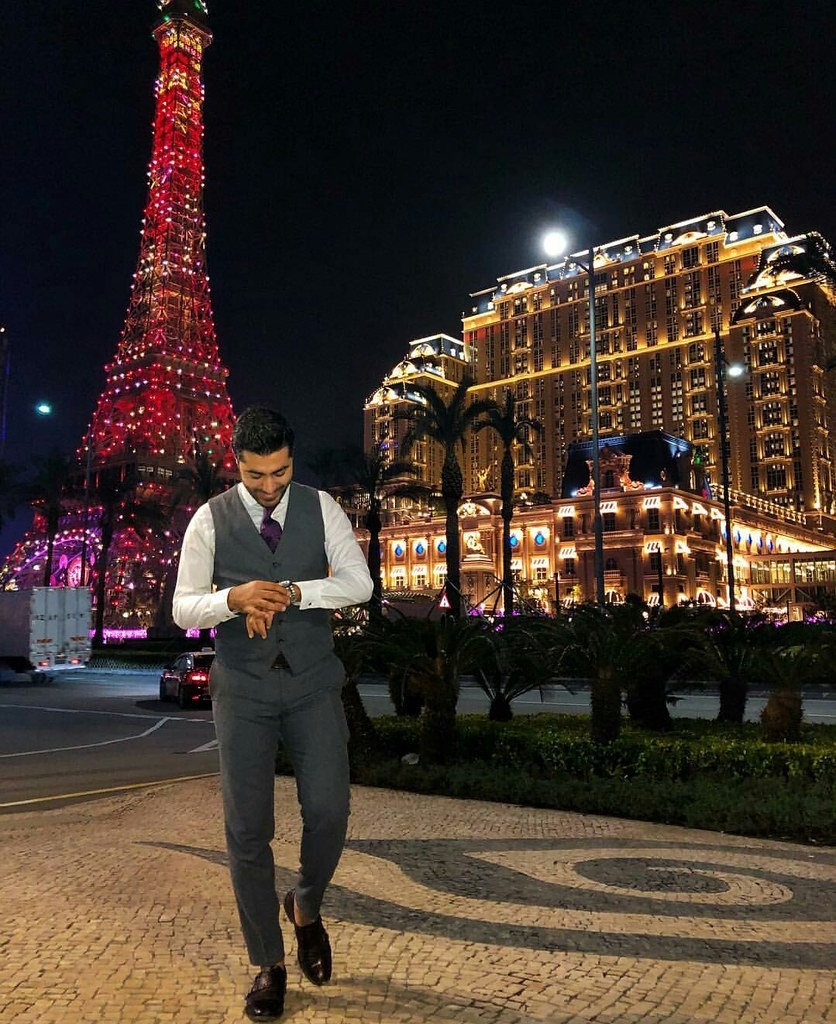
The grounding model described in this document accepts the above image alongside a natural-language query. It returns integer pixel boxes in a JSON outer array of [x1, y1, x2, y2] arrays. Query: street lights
[[34, 401, 93, 586], [712, 325, 748, 610], [531, 222, 604, 608]]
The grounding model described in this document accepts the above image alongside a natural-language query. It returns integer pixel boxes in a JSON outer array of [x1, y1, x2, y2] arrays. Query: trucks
[[0, 588, 92, 686]]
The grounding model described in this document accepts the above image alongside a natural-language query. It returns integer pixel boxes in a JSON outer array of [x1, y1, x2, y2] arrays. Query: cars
[[160, 647, 216, 708]]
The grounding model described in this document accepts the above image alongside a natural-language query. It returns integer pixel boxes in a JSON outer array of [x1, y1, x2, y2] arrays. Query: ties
[[259, 508, 283, 555]]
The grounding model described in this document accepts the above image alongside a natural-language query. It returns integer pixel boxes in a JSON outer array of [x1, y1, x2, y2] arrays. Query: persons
[[172, 408, 374, 1024]]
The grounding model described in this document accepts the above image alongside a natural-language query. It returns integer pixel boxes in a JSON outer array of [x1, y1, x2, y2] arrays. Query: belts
[[271, 654, 290, 670]]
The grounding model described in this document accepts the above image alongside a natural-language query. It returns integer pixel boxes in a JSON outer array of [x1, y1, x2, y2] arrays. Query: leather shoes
[[284, 888, 332, 986], [245, 965, 287, 1022]]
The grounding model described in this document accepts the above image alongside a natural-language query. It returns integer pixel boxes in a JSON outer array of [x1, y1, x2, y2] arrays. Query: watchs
[[278, 579, 298, 605]]
